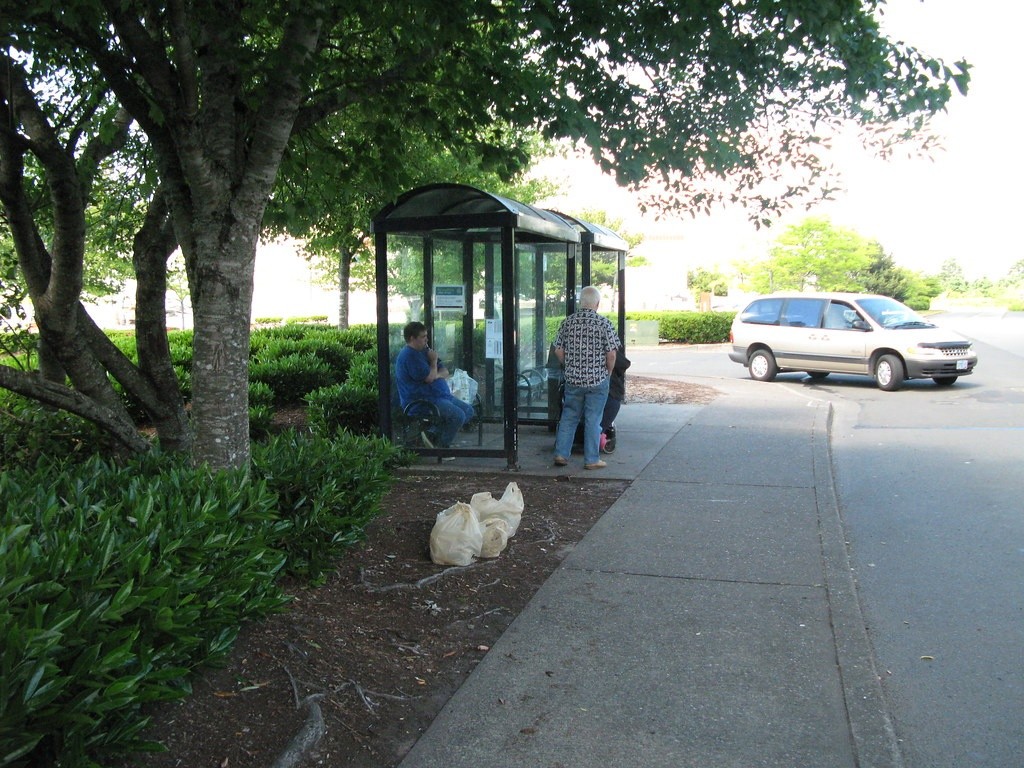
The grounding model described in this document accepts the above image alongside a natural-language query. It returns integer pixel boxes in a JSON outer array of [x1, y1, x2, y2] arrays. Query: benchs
[[401, 391, 484, 465], [500, 369, 545, 418]]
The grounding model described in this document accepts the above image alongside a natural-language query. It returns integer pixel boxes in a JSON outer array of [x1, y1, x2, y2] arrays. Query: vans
[[728, 292, 978, 393]]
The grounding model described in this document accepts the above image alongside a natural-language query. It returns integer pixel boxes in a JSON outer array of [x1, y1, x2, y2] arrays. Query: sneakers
[[420, 430, 455, 460]]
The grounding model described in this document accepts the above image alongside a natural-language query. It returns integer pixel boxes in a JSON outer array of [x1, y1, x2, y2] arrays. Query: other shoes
[[584, 460, 607, 469], [554, 455, 568, 465]]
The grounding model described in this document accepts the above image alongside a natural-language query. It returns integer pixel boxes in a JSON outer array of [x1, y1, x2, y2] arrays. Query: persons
[[554, 286, 619, 471], [395, 320, 475, 461]]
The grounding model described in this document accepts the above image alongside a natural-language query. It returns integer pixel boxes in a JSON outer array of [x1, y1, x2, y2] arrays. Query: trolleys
[[553, 352, 631, 455]]
